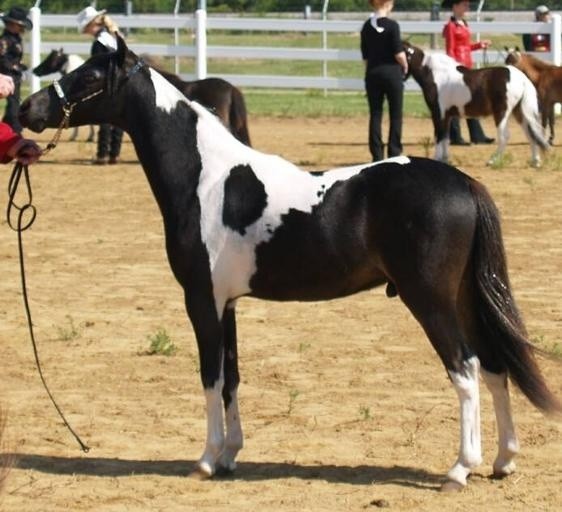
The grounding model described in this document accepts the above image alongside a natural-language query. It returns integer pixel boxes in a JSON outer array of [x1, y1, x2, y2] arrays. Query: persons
[[0, 70, 40, 165], [359, 0, 409, 163], [522, 4, 551, 52], [76, 6, 129, 166], [0, 7, 33, 131], [442, 0, 496, 146]]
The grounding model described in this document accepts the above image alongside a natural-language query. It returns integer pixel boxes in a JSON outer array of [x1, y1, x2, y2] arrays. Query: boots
[[450, 118, 470, 145], [467, 118, 495, 144]]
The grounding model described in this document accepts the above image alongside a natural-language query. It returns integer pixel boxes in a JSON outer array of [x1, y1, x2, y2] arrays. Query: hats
[[1, 6, 35, 30], [534, 5, 551, 17], [74, 5, 106, 35], [442, 0, 478, 8]]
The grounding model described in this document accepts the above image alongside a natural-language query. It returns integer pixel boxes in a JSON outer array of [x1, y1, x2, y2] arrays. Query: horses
[[501, 42, 562, 145], [20, 32, 562, 494], [399, 37, 556, 169], [154, 66, 251, 146], [29, 47, 96, 143]]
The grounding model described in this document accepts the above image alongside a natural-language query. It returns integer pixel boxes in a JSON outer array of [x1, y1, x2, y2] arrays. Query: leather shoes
[[107, 156, 118, 164], [93, 156, 106, 166]]
